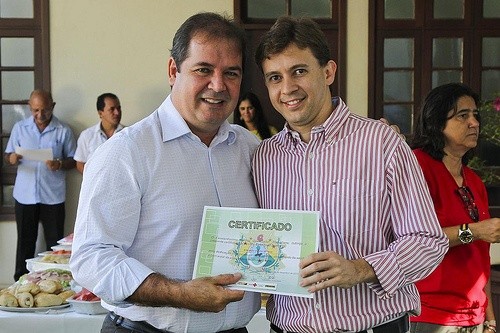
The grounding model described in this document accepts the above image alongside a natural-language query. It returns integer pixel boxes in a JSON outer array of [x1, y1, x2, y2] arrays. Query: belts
[[108, 312, 248, 333], [270, 314, 409, 333]]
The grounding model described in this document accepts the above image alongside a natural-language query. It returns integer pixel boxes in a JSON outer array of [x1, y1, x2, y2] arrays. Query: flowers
[[479, 97, 500, 146]]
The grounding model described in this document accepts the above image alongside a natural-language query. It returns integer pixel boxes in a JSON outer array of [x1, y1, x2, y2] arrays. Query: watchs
[[458, 223, 473, 244]]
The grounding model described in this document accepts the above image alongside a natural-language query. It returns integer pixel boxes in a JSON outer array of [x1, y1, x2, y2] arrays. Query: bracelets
[[483, 320, 496, 332], [57, 158, 64, 169]]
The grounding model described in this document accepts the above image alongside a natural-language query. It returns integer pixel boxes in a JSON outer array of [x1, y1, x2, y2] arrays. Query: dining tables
[[0, 306, 270, 333]]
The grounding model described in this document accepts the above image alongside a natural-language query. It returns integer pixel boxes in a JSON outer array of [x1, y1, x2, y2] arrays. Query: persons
[[409, 82, 500, 333], [68, 12, 407, 333], [235, 95, 279, 143], [4, 88, 77, 282], [73, 93, 130, 174], [249, 15, 449, 333]]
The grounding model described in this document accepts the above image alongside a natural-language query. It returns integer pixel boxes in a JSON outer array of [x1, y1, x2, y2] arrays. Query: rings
[[51, 166, 55, 168]]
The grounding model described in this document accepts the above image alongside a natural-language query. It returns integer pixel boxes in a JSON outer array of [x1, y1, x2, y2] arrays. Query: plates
[[0, 302, 71, 312], [66, 299, 109, 315], [25, 256, 70, 271], [38, 251, 71, 256], [57, 232, 73, 246], [51, 245, 72, 251]]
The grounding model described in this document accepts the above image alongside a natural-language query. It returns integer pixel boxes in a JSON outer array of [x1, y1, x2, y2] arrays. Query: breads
[[0, 279, 76, 308]]
